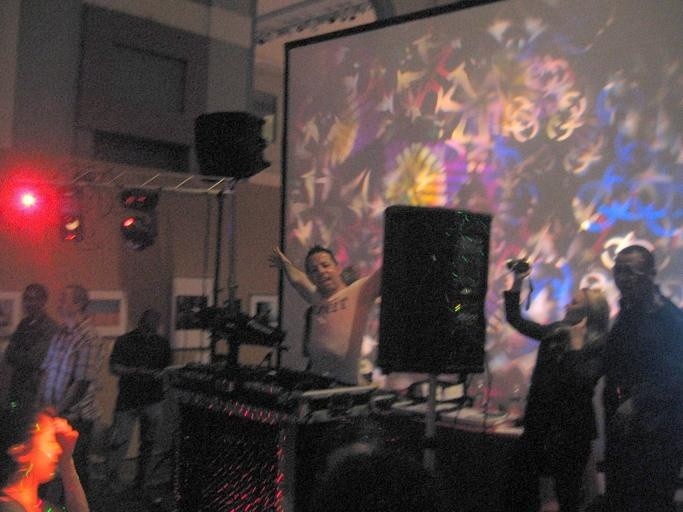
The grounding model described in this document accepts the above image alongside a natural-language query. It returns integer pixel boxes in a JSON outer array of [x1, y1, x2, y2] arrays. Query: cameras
[[507, 259, 530, 273]]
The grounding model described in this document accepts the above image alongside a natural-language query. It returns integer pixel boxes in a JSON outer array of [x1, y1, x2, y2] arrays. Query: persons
[[102, 307, 175, 505], [2, 282, 64, 414], [590, 243, 682, 511], [264, 243, 387, 391], [29, 281, 106, 501], [503, 258, 613, 511], [0, 400, 97, 512]]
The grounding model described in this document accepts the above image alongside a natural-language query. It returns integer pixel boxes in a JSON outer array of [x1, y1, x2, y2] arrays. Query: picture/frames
[[0, 277, 279, 351]]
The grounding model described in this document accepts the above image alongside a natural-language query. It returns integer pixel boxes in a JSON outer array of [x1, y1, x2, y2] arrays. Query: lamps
[[120, 186, 160, 249], [56, 191, 85, 245], [253, 0, 374, 46]]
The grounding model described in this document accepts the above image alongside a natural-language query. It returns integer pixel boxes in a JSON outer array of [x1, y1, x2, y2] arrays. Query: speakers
[[378, 204, 493, 375], [194, 111, 272, 179]]
